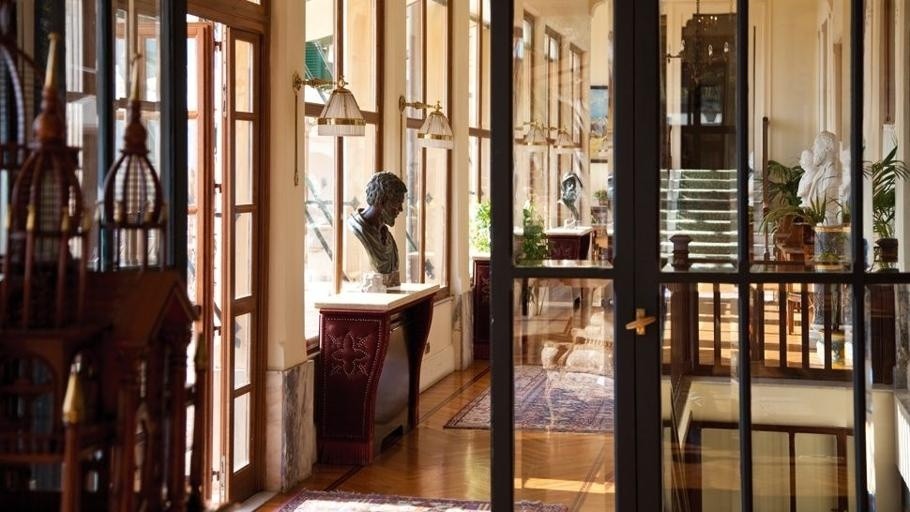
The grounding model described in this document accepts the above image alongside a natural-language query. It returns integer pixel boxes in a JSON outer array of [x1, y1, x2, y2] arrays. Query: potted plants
[[752, 189, 849, 367]]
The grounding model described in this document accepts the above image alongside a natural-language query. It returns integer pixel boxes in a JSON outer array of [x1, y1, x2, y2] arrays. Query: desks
[[471, 254, 492, 361], [516, 227, 593, 260], [316, 284, 439, 464]]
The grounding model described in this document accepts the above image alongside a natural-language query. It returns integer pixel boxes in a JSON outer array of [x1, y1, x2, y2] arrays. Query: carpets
[[441, 363, 614, 435], [282, 487, 571, 511]]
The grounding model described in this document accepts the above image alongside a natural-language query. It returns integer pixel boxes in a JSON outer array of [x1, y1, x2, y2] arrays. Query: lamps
[[590, 135, 608, 157], [513, 120, 579, 156], [398, 94, 457, 149], [0, 0, 168, 235], [287, 72, 368, 139]]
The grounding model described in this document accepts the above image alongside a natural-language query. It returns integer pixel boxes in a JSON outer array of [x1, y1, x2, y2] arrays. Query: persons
[[345, 171, 410, 288], [808, 128, 844, 210], [796, 148, 819, 197]]
